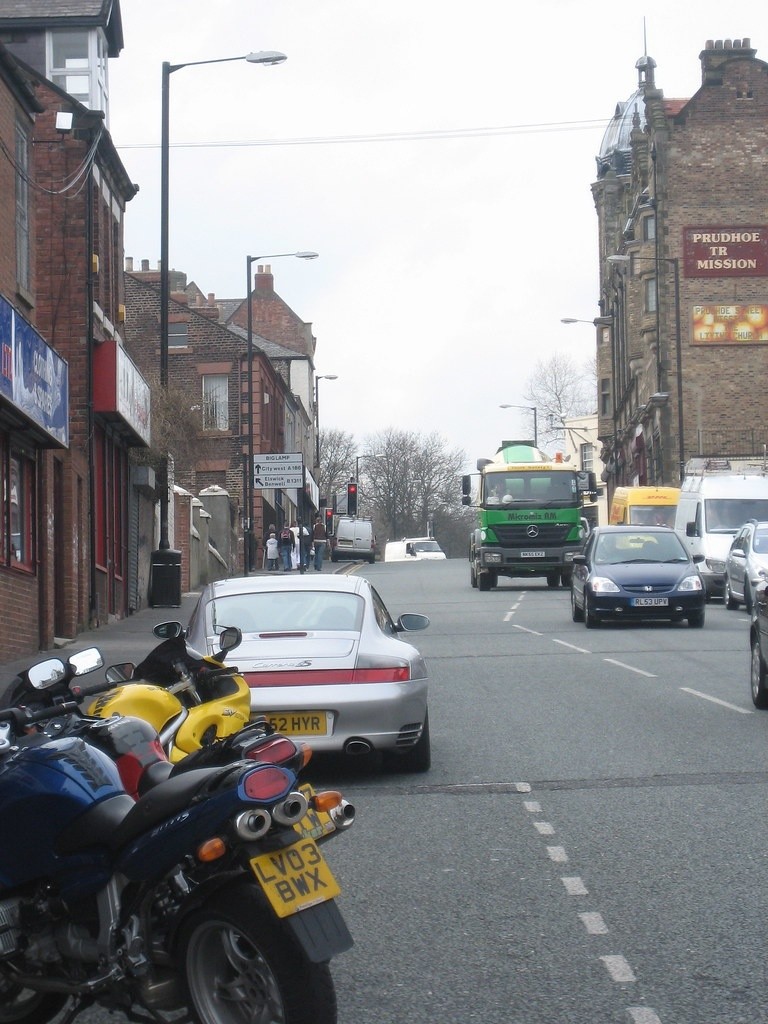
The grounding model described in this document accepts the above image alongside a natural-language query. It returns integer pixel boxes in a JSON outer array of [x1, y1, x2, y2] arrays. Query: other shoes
[[270, 564, 322, 572]]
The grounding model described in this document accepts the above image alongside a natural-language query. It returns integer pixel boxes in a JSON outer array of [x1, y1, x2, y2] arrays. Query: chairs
[[489, 476, 507, 500], [546, 474, 573, 501]]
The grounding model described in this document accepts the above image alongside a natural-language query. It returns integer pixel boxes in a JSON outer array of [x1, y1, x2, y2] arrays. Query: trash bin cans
[[150, 549, 182, 605]]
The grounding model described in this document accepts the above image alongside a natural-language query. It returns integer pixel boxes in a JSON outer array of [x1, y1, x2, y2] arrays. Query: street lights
[[606, 254, 687, 485], [145, 50, 289, 607], [245, 249, 321, 565], [313, 372, 341, 473], [498, 403, 539, 448], [356, 454, 386, 484], [560, 317, 618, 485]]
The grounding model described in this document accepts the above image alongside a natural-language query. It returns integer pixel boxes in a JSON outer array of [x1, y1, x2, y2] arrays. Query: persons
[[488, 479, 513, 502], [262, 524, 282, 571], [596, 534, 624, 563], [289, 516, 331, 571], [277, 521, 295, 571]]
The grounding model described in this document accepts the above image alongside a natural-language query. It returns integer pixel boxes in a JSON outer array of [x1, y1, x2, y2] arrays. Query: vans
[[579, 517, 591, 540], [609, 486, 682, 552], [673, 458, 768, 604], [384, 537, 448, 561], [330, 515, 376, 564]]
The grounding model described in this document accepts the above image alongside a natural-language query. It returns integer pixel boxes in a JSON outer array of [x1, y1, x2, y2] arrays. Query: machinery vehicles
[[462, 439, 599, 592]]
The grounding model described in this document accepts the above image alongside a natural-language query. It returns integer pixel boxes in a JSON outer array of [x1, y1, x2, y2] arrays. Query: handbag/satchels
[[281, 529, 291, 541], [302, 527, 309, 537]]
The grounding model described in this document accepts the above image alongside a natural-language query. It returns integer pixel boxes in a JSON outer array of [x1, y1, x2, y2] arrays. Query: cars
[[749, 579, 768, 711], [570, 524, 707, 627]]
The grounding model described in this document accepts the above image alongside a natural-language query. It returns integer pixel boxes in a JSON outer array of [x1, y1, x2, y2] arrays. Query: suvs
[[724, 517, 768, 616]]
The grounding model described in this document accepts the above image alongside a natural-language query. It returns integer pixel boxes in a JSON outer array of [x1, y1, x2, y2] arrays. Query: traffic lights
[[347, 483, 358, 515], [324, 508, 334, 536]]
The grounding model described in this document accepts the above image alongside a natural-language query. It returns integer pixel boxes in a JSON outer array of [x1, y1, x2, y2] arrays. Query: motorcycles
[[0, 620, 355, 1024]]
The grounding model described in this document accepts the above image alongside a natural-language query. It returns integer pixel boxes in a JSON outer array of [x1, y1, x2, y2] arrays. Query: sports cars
[[152, 574, 431, 774]]
